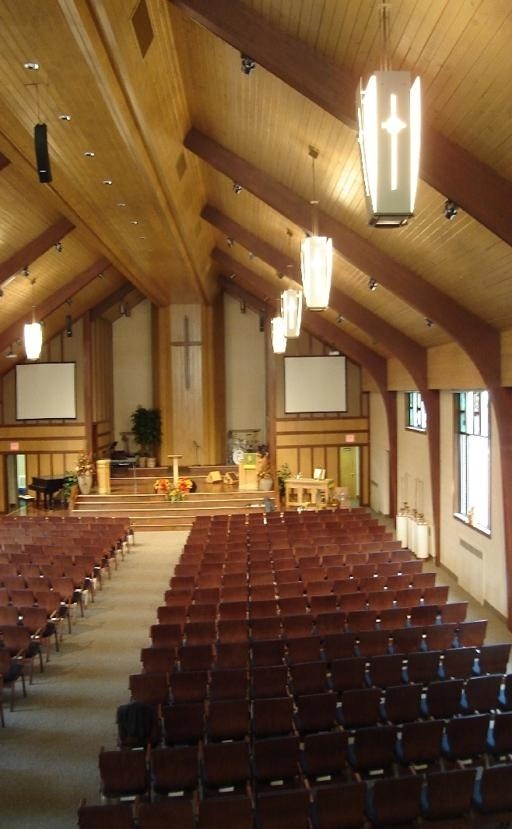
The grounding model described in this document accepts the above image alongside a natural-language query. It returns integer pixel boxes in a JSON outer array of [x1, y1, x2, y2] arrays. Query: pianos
[[26, 475, 70, 510]]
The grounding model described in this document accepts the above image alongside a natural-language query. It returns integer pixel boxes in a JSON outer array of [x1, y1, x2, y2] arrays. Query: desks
[[284, 477, 334, 509]]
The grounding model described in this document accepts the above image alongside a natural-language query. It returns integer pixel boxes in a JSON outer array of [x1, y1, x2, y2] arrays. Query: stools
[[18, 495, 35, 515]]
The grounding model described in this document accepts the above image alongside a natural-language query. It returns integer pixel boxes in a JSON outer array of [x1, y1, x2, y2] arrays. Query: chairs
[[308, 469, 327, 503]]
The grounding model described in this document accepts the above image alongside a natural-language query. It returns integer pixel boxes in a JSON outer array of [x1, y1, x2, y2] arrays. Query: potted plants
[[144, 408, 162, 468], [129, 408, 148, 468]]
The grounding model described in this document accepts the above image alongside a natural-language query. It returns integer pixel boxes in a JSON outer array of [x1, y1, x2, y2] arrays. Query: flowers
[[153, 479, 193, 502], [75, 455, 95, 477]]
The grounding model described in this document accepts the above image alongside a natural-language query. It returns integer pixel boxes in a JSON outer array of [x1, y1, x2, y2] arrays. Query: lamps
[[271, 276, 288, 354], [280, 233, 303, 339], [33, 80, 53, 185], [66, 298, 73, 337], [240, 290, 245, 315], [300, 150, 334, 311], [24, 276, 43, 361], [355, 2, 423, 228], [259, 310, 265, 333]]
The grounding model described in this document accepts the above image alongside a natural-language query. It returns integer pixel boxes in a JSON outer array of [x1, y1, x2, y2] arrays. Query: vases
[[260, 477, 273, 491], [76, 472, 93, 494]]
